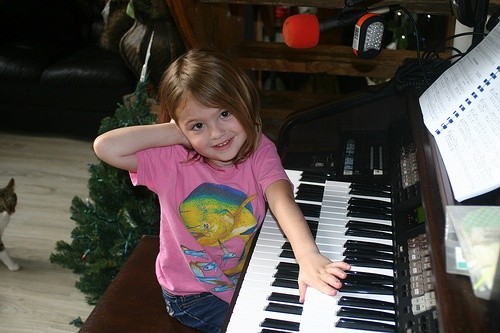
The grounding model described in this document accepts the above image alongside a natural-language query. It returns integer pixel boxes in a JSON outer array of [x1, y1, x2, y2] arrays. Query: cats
[[0, 178, 21, 271]]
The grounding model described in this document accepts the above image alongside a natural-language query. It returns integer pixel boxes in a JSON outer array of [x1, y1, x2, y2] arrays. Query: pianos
[[222, 57, 500, 333]]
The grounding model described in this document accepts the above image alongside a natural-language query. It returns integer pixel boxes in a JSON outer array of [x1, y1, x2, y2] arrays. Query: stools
[[77, 236, 197, 333]]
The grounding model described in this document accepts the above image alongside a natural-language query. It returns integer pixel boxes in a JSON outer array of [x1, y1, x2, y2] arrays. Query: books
[[419, 22, 500, 202]]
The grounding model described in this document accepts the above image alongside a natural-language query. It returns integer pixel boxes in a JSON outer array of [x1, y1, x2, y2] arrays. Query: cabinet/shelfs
[[167, 0, 456, 155]]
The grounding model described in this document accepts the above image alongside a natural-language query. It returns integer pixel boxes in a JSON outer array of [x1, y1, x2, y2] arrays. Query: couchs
[[0, 0, 168, 146]]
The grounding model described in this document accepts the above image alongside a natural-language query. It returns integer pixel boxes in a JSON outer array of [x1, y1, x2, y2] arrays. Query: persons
[[93, 48, 351, 333]]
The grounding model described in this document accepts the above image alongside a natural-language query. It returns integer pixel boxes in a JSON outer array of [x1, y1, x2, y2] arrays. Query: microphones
[[283, 4, 399, 49]]
